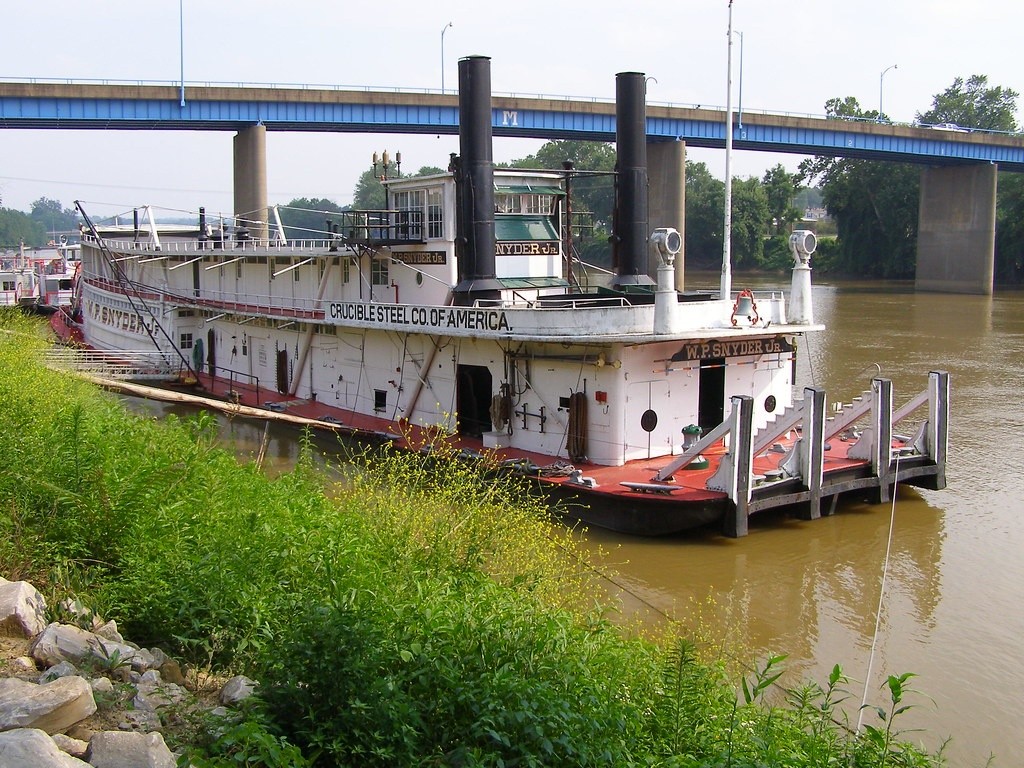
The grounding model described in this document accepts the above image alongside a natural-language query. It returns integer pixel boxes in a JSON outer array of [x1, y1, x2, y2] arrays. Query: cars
[[926, 123, 970, 133]]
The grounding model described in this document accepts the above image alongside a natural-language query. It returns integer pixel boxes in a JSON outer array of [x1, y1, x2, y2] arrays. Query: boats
[[52, 146, 952, 540]]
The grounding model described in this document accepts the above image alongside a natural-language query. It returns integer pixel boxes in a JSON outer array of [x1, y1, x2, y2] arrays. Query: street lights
[[880, 64, 898, 123], [441, 21, 453, 93]]
